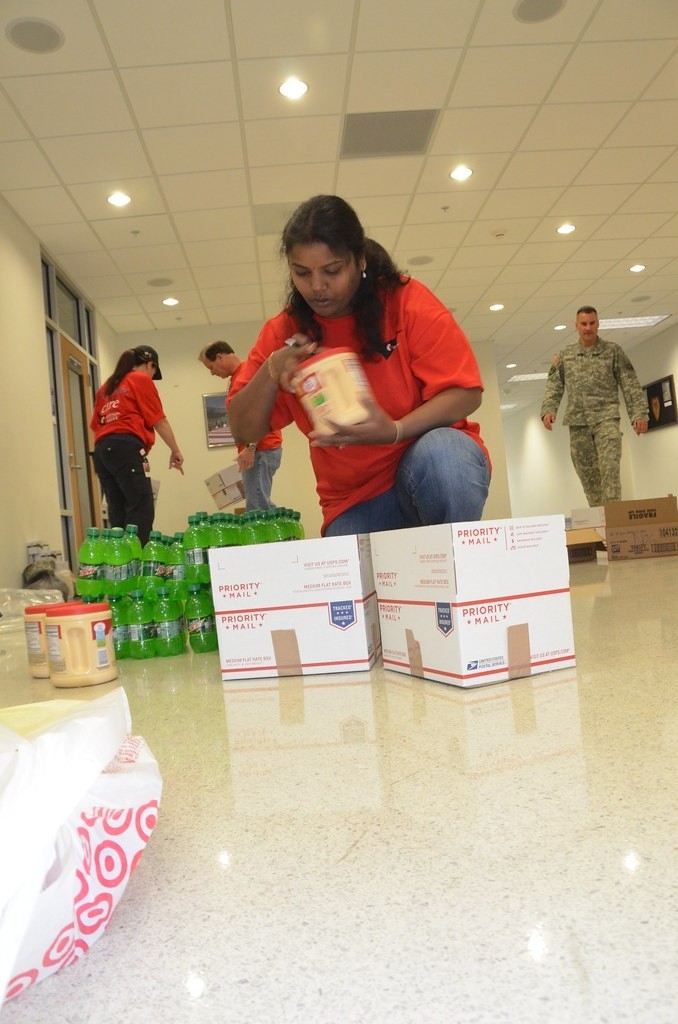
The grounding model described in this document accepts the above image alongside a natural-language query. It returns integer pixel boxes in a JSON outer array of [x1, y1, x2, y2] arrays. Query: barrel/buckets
[[289, 344, 371, 439], [24, 602, 83, 677], [46, 605, 118, 688]]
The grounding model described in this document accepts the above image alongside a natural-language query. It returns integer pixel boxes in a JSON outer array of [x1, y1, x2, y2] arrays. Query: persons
[[199, 341, 283, 513], [540, 306, 649, 508], [88, 345, 184, 549], [225, 194, 491, 538]]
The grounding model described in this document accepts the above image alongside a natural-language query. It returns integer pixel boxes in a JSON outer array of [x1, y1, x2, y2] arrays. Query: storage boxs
[[204, 462, 246, 512], [25, 540, 68, 573], [206, 495, 678, 690]]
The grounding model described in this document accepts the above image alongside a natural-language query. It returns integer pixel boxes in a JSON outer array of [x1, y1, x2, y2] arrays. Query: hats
[[135, 345, 163, 381]]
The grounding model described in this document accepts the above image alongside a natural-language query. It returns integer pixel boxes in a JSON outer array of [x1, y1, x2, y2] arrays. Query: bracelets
[[268, 350, 281, 380], [392, 421, 403, 445]]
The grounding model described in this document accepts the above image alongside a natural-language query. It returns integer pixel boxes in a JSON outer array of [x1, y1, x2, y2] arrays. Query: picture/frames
[[642, 374, 678, 434], [201, 390, 237, 449]]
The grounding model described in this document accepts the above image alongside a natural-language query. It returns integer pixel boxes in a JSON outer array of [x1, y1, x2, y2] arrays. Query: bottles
[[76, 506, 305, 659]]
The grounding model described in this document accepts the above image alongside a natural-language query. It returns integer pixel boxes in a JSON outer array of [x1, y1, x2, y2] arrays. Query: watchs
[[246, 443, 257, 450]]
